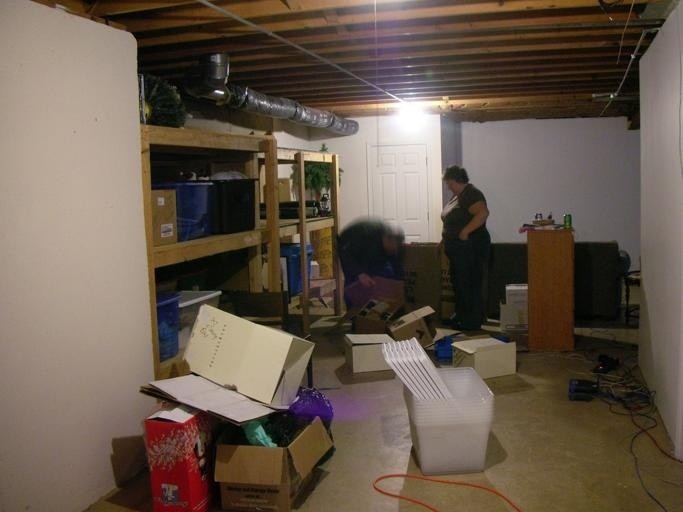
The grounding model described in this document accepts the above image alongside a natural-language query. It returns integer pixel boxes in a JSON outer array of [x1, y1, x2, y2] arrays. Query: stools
[[624, 270, 641, 326]]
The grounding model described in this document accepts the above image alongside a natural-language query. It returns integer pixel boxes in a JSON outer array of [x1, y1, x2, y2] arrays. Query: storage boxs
[[346, 284, 528, 476], [142, 405, 335, 512]]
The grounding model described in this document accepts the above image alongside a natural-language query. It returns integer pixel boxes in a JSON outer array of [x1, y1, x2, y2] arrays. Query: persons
[[439, 163, 494, 333], [335, 219, 407, 335]]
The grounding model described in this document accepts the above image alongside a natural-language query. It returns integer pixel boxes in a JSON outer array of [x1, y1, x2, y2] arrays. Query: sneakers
[[443, 313, 481, 330]]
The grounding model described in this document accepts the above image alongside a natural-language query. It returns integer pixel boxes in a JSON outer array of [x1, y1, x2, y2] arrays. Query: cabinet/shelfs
[[526, 229, 575, 353]]
